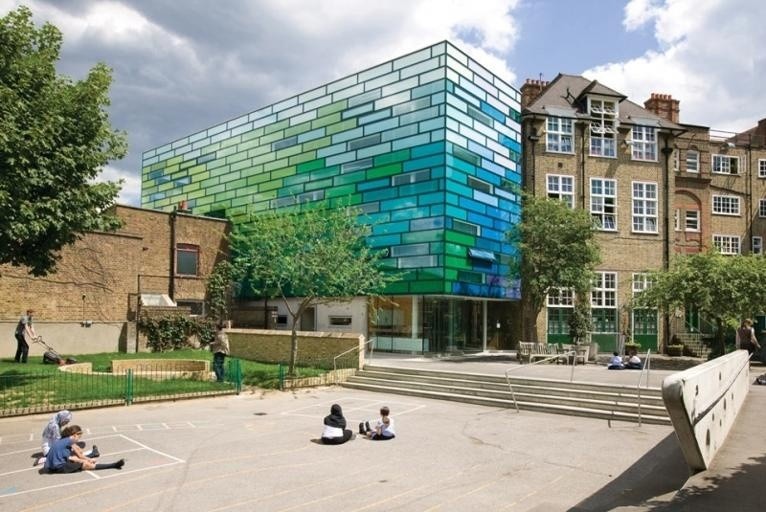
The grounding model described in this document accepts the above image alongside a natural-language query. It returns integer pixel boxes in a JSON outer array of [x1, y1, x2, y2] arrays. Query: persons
[[45, 425, 125, 473], [15, 309, 39, 363], [623, 350, 642, 369], [209, 324, 230, 381], [607, 352, 624, 367], [736, 318, 761, 368], [322, 405, 352, 444], [359, 407, 396, 440], [42, 409, 86, 457]]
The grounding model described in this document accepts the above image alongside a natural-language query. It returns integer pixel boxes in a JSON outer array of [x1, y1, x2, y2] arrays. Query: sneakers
[[359, 422, 370, 434], [117, 460, 123, 468], [93, 445, 99, 456]]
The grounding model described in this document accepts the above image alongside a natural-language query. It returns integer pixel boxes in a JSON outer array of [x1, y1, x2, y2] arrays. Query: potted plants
[[625, 342, 641, 355], [666, 344, 684, 356]]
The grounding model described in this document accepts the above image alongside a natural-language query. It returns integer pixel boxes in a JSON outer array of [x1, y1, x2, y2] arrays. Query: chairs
[[517, 341, 586, 365]]
